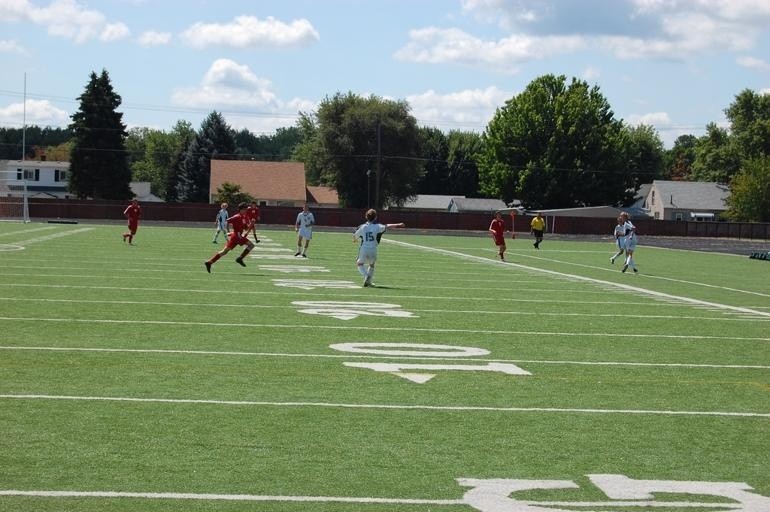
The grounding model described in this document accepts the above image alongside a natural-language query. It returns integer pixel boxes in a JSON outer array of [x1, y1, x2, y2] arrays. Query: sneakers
[[295, 252, 306, 257], [124, 235, 134, 246], [364, 274, 375, 287], [205, 240, 260, 273], [499, 242, 639, 274]]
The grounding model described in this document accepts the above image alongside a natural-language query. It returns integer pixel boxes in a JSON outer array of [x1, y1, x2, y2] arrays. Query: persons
[[204, 202, 255, 273], [353, 208, 406, 286], [619, 211, 638, 272], [122, 198, 143, 245], [213, 202, 232, 244], [488, 211, 507, 261], [531, 212, 545, 249], [609, 216, 625, 266], [295, 203, 315, 258], [245, 201, 261, 242]]
